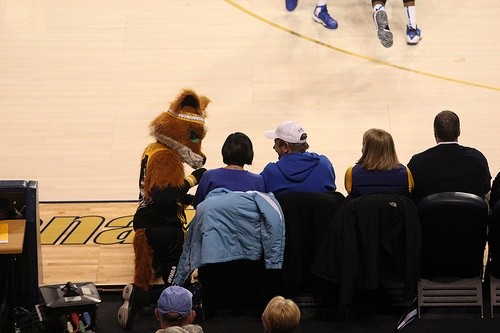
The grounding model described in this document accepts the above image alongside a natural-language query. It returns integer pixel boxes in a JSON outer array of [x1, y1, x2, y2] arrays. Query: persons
[[192, 132, 265, 209], [372, 0, 422, 48], [260, 120, 336, 194], [262, 297, 301, 333], [345, 128, 415, 197], [406, 110, 492, 200], [285, 0, 339, 29], [155, 285, 204, 333]]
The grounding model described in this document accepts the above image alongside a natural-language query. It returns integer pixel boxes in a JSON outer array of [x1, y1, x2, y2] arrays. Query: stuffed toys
[[116, 86, 210, 329]]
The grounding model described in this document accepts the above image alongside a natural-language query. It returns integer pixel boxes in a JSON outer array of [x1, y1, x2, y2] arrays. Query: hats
[[265, 121, 308, 144], [158, 286, 193, 318]]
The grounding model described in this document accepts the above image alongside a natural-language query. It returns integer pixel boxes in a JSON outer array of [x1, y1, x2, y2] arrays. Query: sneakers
[[373, 4, 393, 48], [286, 0, 297, 11], [405, 24, 423, 45], [313, 3, 338, 29]]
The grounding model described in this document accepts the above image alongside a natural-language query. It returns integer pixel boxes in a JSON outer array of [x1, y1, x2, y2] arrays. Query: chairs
[[274, 187, 346, 307], [334, 190, 422, 322], [488, 199, 500, 317], [413, 191, 491, 319], [200, 257, 273, 319]]
